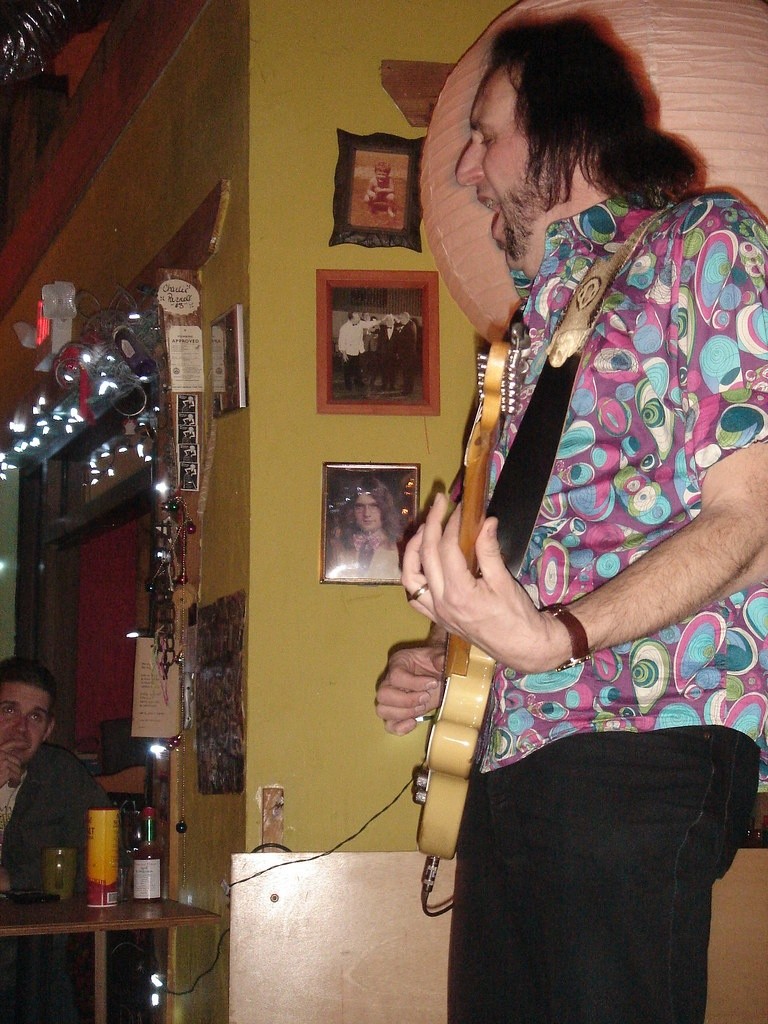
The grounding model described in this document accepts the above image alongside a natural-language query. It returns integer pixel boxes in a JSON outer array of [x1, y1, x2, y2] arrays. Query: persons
[[328, 476, 409, 579], [0, 657, 132, 1024], [338, 310, 417, 395], [374, 13, 768, 1024], [364, 162, 396, 218]]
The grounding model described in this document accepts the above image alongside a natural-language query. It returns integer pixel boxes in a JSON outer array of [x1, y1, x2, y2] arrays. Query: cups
[[41, 847, 76, 898], [116, 867, 127, 902]]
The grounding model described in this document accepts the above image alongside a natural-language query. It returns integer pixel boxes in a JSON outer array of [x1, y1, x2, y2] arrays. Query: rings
[[412, 583, 429, 600]]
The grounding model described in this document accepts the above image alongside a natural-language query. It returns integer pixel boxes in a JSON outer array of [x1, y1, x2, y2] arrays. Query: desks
[[0, 891, 221, 1024]]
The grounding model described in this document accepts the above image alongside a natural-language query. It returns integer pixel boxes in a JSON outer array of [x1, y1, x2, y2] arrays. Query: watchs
[[547, 602, 592, 671]]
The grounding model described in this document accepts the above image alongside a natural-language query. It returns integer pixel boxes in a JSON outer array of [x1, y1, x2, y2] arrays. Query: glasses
[[353, 503, 382, 513]]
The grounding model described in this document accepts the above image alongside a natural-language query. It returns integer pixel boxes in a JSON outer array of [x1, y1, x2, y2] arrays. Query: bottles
[[132, 807, 163, 900]]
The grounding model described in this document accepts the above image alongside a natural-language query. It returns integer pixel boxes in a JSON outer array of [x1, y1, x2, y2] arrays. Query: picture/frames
[[320, 459, 420, 588], [328, 127, 428, 253], [314, 265, 416, 423]]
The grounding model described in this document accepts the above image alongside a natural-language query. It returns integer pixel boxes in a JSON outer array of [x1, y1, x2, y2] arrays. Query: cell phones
[[7, 890, 61, 902]]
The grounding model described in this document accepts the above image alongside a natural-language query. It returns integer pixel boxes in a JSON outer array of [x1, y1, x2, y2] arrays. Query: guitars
[[413, 337, 521, 858]]
